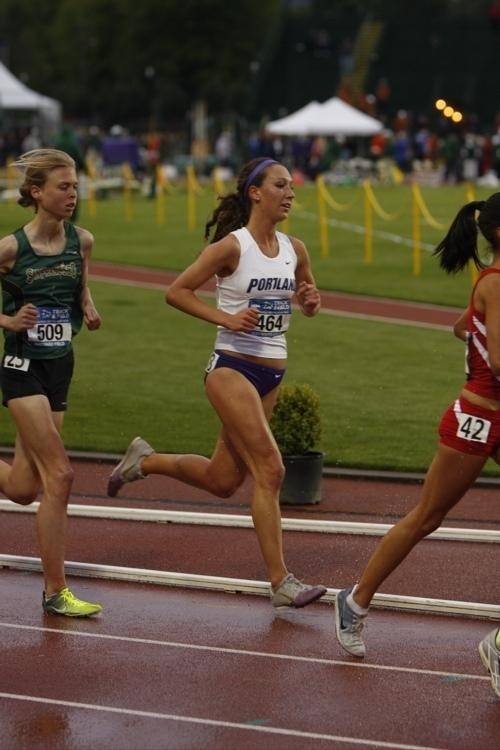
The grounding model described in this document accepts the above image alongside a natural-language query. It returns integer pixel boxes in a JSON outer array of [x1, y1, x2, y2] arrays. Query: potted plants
[[266, 379, 327, 508]]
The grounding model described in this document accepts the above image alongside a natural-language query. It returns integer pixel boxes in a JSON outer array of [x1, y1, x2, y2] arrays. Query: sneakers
[[269, 572, 328, 608], [106, 436, 155, 497], [42, 586, 103, 618], [478, 627, 500, 698], [334, 587, 368, 658]]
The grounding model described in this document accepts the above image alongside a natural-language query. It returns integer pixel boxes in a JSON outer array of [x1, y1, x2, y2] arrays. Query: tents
[[267, 97, 384, 136]]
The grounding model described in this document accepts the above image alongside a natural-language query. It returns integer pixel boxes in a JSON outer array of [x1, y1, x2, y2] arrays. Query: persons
[[214, 124, 500, 186], [0, 149, 104, 619], [107, 156, 326, 608], [334, 192, 499, 696], [1, 124, 145, 175]]
